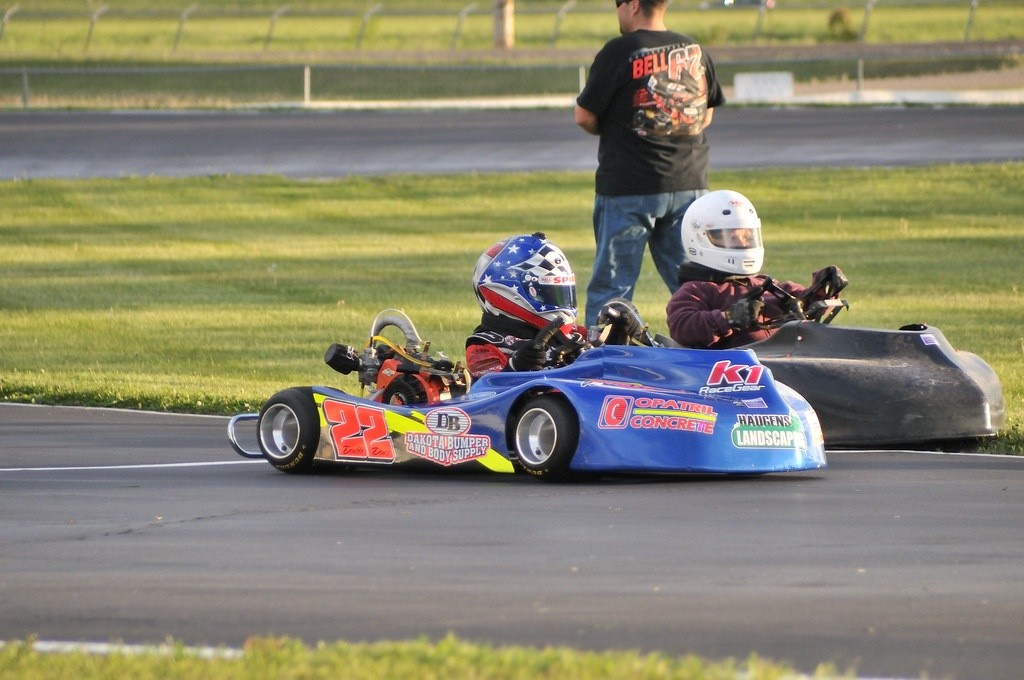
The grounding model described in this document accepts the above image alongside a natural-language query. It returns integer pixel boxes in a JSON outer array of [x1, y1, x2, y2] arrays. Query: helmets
[[681, 188, 765, 275], [470, 235, 577, 334]]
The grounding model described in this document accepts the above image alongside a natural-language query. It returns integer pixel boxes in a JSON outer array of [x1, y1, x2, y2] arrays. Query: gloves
[[727, 285, 765, 325], [603, 307, 640, 346], [811, 265, 848, 300], [512, 340, 549, 371]]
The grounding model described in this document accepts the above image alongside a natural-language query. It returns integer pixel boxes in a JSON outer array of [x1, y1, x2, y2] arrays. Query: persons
[[574, 0, 727, 328], [465, 232, 643, 387], [665, 189, 847, 351]]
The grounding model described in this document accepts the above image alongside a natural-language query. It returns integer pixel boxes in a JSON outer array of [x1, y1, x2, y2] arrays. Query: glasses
[[616, 0, 623, 8]]
